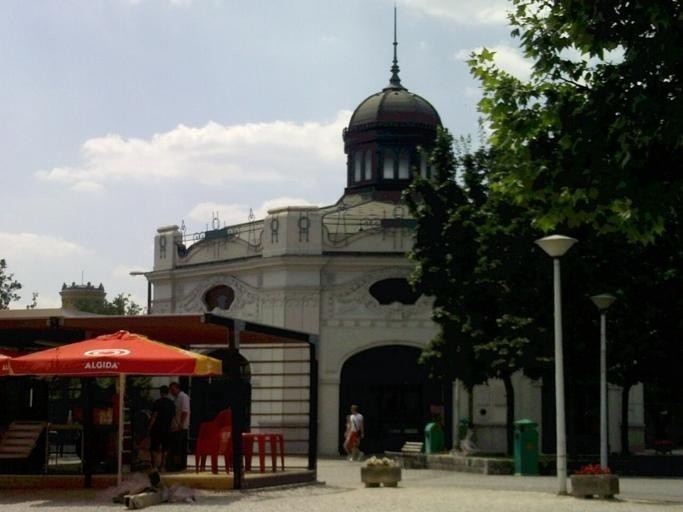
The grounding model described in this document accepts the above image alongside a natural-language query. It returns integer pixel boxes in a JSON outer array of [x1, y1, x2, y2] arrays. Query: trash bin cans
[[512, 418, 540, 477], [424, 422, 445, 455]]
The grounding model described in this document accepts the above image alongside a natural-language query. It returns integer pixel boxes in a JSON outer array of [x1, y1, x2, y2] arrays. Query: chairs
[[194, 408, 233, 475]]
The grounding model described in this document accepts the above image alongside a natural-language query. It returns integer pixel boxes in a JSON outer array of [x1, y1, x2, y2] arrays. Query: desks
[[240, 431, 286, 474]]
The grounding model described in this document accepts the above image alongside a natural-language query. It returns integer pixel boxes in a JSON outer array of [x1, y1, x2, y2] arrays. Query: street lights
[[533, 233, 579, 496], [587, 291, 619, 473], [129, 271, 152, 314]]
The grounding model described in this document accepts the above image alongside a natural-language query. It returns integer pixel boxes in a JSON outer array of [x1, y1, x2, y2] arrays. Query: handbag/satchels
[[357, 429, 361, 437], [170, 416, 179, 433]]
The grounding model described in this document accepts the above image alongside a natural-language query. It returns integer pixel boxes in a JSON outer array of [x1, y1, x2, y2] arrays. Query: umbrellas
[[8, 329, 222, 484]]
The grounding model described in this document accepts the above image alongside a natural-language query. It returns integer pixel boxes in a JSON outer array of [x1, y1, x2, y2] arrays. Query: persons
[[166, 380, 191, 474], [132, 386, 155, 444], [342, 409, 353, 460], [347, 403, 367, 460], [147, 385, 177, 475]]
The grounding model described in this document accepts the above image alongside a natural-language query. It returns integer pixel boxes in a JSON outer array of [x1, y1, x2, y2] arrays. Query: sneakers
[[358, 452, 364, 461]]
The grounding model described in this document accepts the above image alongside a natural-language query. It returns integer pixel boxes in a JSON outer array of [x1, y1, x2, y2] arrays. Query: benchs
[[383, 441, 424, 469]]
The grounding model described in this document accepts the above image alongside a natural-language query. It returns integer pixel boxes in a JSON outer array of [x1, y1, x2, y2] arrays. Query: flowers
[[577, 462, 611, 474], [360, 456, 400, 466]]
